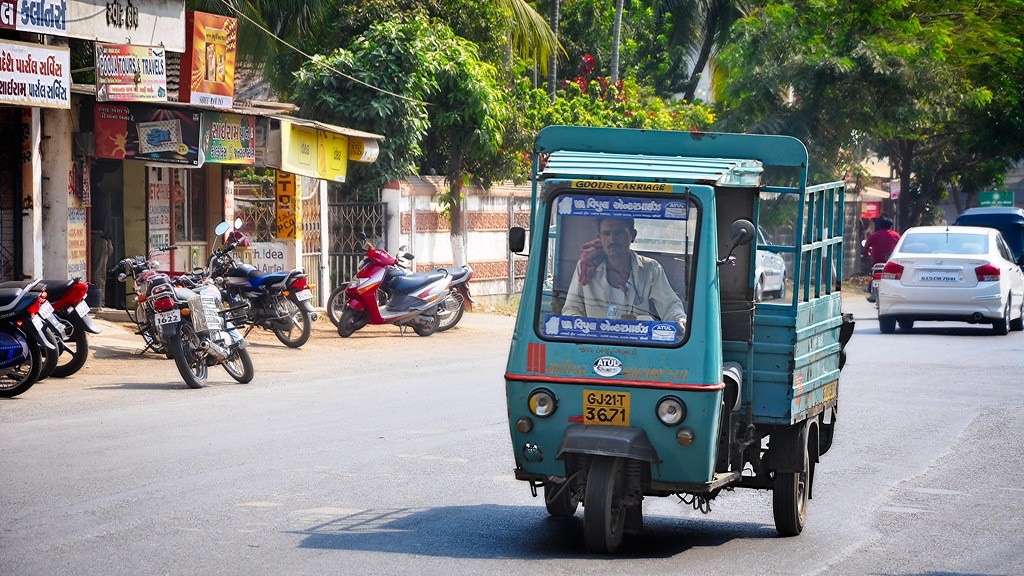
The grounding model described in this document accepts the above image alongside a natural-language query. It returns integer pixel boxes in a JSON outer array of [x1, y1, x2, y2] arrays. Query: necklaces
[[608, 258, 641, 303]]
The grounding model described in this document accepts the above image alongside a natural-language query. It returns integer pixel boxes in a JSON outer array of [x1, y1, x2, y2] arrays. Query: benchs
[[903, 243, 982, 255]]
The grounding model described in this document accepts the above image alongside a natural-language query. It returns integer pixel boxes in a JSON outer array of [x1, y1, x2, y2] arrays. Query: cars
[[878, 226, 1024, 334]]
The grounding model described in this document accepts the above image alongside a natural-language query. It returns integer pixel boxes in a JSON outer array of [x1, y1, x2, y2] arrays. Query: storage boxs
[[187, 296, 222, 333]]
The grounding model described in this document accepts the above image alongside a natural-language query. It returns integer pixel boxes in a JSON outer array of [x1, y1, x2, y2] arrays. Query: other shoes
[[867, 296, 876, 302]]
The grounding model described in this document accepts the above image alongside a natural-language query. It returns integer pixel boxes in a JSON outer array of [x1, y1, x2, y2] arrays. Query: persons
[[561, 218, 686, 334], [863, 218, 901, 303], [936, 214, 947, 226], [89, 167, 113, 307]]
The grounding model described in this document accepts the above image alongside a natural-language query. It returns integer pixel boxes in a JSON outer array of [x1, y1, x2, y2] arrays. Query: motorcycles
[[108, 245, 255, 389], [0, 277, 102, 398], [861, 239, 887, 303], [953, 207, 1024, 266], [327, 232, 474, 338], [205, 217, 318, 347], [503, 125, 854, 553]]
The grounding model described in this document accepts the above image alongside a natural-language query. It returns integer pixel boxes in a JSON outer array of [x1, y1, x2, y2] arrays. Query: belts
[[92, 230, 104, 234]]
